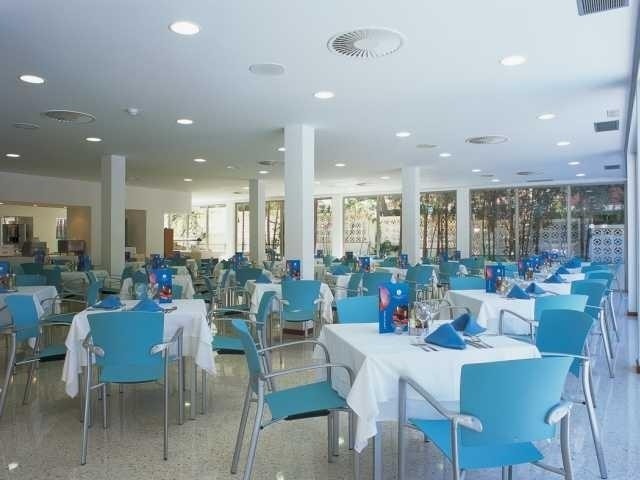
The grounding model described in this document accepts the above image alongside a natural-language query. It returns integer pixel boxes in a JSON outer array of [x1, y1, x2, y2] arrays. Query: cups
[[8, 273, 16, 291]]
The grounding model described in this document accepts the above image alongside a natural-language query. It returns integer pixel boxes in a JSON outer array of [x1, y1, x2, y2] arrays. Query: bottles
[[496, 276, 502, 295], [527, 268, 534, 281]]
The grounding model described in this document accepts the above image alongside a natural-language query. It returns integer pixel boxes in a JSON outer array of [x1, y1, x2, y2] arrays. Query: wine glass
[[426, 301, 439, 330], [502, 277, 509, 298], [414, 303, 431, 340]]
